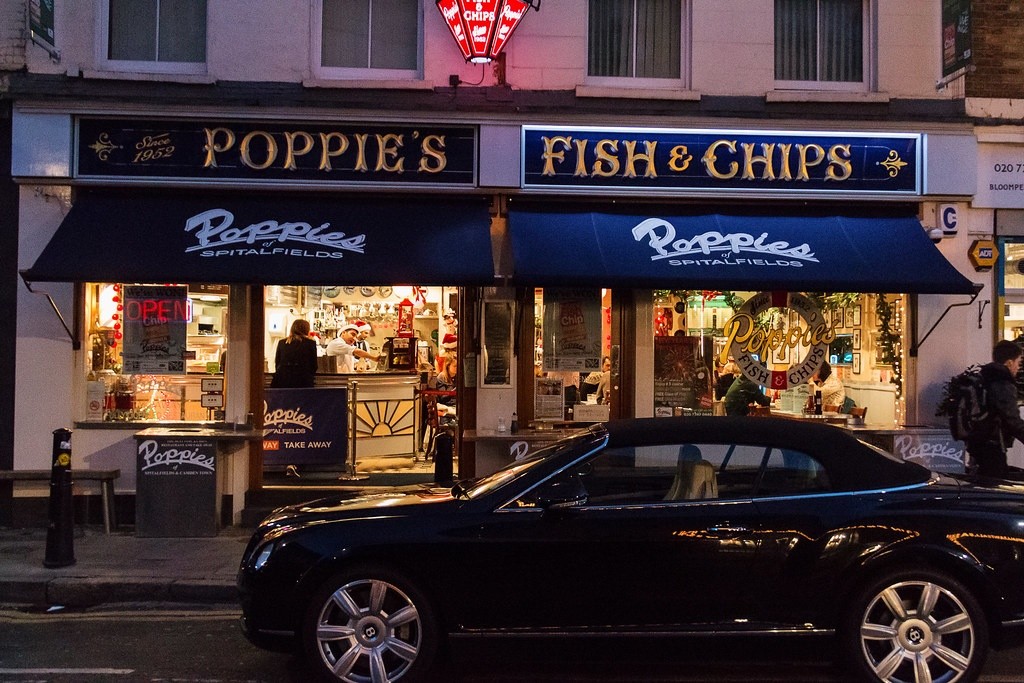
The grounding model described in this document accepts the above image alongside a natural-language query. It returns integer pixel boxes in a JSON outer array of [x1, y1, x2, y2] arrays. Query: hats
[[337, 324, 360, 337], [355, 321, 372, 335], [442, 333, 458, 348]]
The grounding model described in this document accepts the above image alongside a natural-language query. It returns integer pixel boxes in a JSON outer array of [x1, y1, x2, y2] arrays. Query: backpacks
[[949, 373, 995, 441]]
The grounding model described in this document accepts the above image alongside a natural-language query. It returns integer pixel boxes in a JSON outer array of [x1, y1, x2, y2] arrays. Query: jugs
[[197, 316, 219, 335]]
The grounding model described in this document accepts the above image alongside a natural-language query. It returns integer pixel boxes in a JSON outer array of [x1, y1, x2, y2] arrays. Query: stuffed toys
[[442, 309, 458, 349]]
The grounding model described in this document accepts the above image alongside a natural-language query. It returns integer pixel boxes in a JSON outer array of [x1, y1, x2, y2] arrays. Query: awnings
[[21, 190, 494, 285], [509, 195, 978, 295]]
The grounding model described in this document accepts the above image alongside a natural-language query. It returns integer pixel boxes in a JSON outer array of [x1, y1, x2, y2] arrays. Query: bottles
[[830, 348, 838, 365], [807, 396, 814, 412], [379, 353, 387, 366], [511, 412, 518, 434]]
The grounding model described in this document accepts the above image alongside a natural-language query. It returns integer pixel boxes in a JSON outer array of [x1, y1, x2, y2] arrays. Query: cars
[[234, 414, 1024, 683]]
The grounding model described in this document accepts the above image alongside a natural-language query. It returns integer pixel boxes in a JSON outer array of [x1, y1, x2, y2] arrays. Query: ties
[[358, 342, 362, 348]]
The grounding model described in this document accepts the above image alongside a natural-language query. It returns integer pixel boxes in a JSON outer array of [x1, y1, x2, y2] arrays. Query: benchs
[[0, 468, 123, 536]]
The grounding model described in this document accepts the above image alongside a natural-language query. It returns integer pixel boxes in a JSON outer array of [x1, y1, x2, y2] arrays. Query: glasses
[[446, 348, 456, 352]]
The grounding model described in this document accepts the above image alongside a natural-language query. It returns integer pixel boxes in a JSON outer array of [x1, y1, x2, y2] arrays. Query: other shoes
[[286, 465, 300, 478]]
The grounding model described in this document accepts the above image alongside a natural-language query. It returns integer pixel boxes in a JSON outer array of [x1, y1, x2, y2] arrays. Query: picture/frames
[[820, 304, 862, 375]]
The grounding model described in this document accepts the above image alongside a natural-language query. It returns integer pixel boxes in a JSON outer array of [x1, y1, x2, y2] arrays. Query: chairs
[[660, 442, 701, 502], [745, 403, 867, 427], [684, 458, 719, 501], [415, 387, 458, 460]]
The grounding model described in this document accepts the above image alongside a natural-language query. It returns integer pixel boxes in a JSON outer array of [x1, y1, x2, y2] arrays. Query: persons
[[814, 361, 845, 411], [965, 340, 1024, 480], [352, 321, 371, 370], [326, 324, 386, 372], [270, 319, 318, 388], [432, 353, 457, 463], [580, 357, 610, 405], [714, 356, 771, 416]]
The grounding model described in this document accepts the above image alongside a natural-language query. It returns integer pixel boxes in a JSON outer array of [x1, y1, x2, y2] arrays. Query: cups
[[793, 395, 806, 413], [587, 394, 595, 403], [781, 394, 793, 411], [498, 417, 505, 431]]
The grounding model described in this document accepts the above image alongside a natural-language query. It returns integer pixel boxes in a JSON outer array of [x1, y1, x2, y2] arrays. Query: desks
[[769, 410, 852, 427], [132, 427, 276, 538]]
